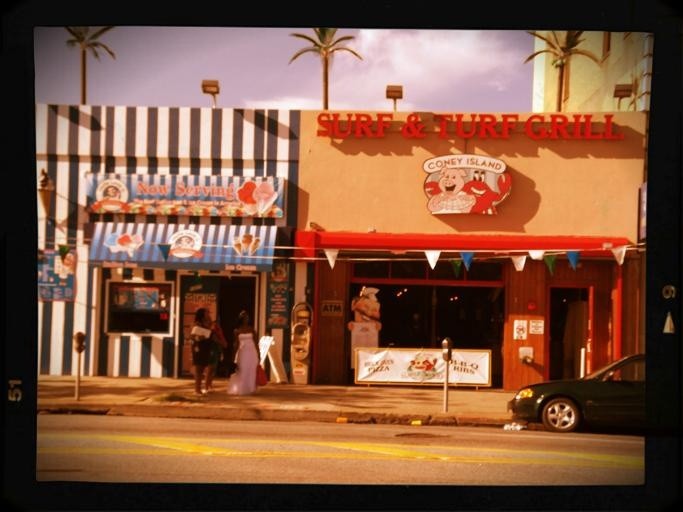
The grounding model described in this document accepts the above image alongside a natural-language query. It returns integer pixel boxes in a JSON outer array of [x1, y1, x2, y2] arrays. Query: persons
[[103, 186, 121, 201], [227, 310, 262, 395], [190, 308, 225, 397], [56, 253, 74, 286]]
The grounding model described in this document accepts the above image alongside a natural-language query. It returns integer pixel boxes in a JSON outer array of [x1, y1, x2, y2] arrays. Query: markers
[[252, 182, 278, 216], [251, 239, 261, 254], [242, 233, 253, 253], [232, 238, 242, 256], [237, 182, 255, 215]]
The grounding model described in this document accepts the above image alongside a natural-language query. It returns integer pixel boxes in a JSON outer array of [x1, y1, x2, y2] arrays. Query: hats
[[512, 352, 645, 432]]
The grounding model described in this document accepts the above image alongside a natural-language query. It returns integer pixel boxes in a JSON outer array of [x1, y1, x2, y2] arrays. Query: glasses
[[613, 83, 633, 111], [201, 79, 220, 107], [386, 85, 403, 112]]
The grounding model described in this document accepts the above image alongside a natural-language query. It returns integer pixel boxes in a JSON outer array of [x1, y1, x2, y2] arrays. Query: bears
[[74, 332, 85, 400], [442, 338, 451, 414]]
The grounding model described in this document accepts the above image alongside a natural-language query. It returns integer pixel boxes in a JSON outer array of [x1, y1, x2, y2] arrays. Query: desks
[[386, 85, 402, 111], [202, 80, 219, 107], [613, 84, 632, 111]]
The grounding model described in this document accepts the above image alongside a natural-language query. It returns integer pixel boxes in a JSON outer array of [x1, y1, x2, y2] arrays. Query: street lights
[[256, 364, 267, 386]]
